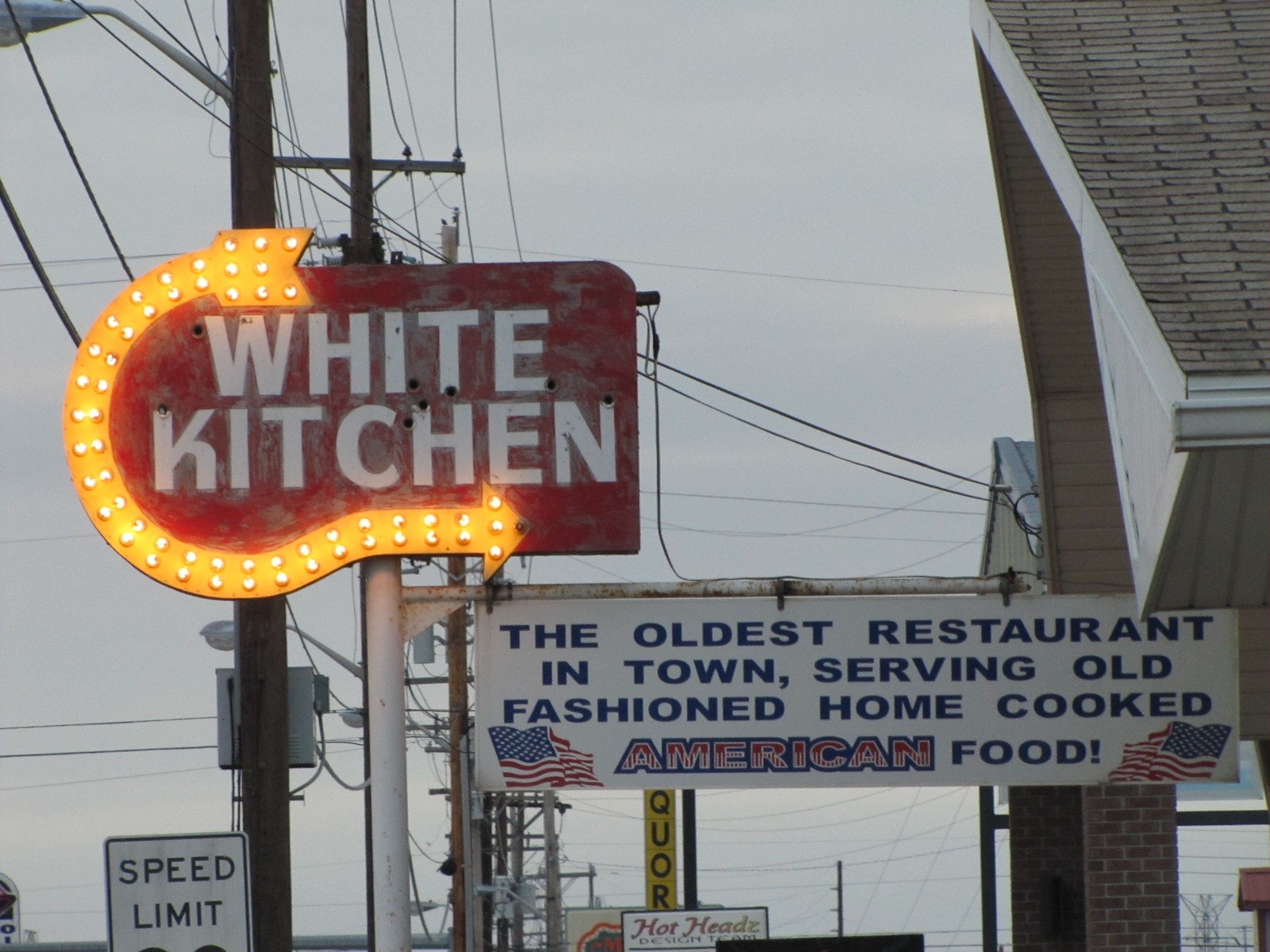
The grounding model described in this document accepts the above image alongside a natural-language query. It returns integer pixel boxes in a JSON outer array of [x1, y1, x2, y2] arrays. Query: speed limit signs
[[105, 832, 253, 952]]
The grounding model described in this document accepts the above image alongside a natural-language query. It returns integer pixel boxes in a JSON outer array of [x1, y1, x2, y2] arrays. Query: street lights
[[0, 2, 291, 952], [198, 621, 468, 952]]
